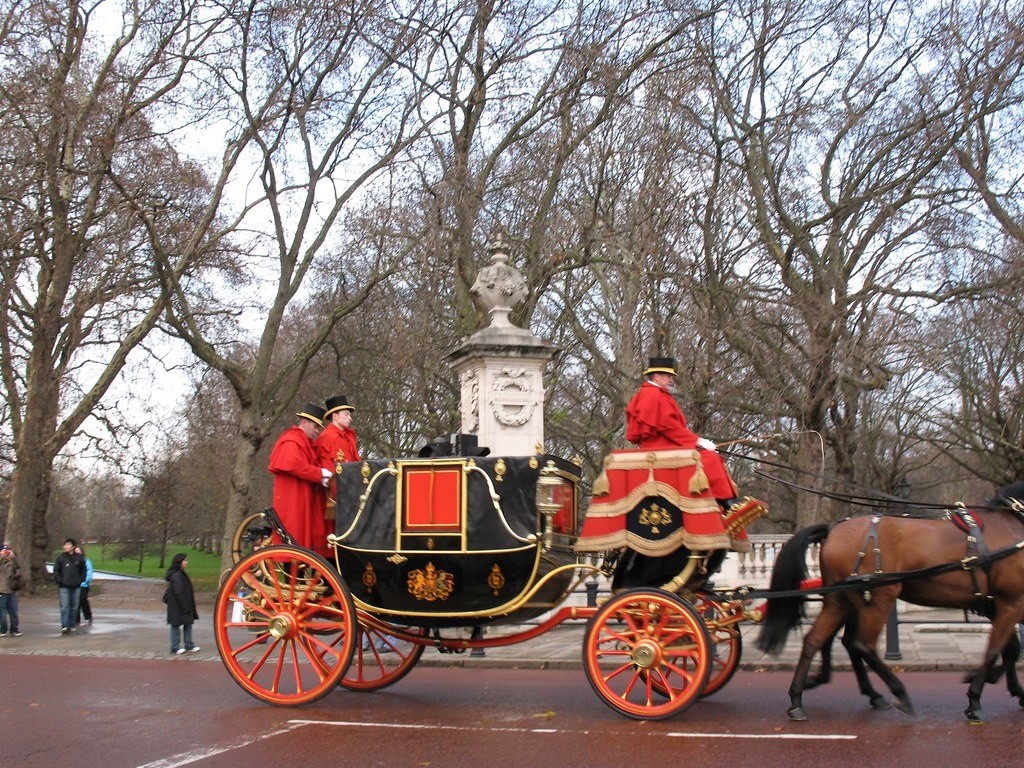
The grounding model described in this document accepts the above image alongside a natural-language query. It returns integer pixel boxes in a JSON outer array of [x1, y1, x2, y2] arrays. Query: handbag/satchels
[[9, 575, 23, 590], [162, 590, 169, 602]]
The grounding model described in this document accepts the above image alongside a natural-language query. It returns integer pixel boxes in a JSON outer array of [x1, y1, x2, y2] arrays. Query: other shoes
[[176, 648, 185, 654], [190, 647, 200, 652], [13, 631, 21, 637], [719, 497, 738, 510], [62, 627, 68, 631], [1, 632, 6, 636], [71, 627, 76, 631], [704, 580, 715, 589], [84, 620, 90, 625]]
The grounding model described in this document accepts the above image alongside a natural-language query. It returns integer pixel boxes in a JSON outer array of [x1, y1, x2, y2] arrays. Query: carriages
[[212, 432, 1024, 725]]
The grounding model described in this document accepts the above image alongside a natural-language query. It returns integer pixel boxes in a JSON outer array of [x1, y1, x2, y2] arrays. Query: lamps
[[534, 460, 563, 552]]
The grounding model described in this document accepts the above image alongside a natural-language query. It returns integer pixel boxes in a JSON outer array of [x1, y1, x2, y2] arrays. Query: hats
[[642, 357, 678, 376], [324, 395, 355, 419], [296, 403, 326, 428], [0, 545, 9, 551]]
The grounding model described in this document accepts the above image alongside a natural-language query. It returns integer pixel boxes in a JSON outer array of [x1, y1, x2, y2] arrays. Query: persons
[[53, 539, 87, 633], [0, 545, 22, 637], [362, 622, 398, 653], [267, 403, 333, 585], [313, 395, 362, 502], [248, 535, 270, 644], [164, 553, 200, 655], [418, 446, 434, 458], [624, 358, 736, 511], [74, 547, 93, 625]]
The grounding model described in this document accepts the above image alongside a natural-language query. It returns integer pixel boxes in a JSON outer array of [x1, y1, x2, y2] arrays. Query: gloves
[[322, 477, 330, 487], [321, 468, 333, 478], [697, 438, 716, 451]]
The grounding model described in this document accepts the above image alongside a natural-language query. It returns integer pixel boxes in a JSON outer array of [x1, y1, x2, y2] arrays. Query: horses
[[752, 483, 1024, 721]]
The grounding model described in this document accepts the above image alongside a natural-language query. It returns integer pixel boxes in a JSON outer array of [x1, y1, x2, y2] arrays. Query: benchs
[[576, 447, 771, 556]]
